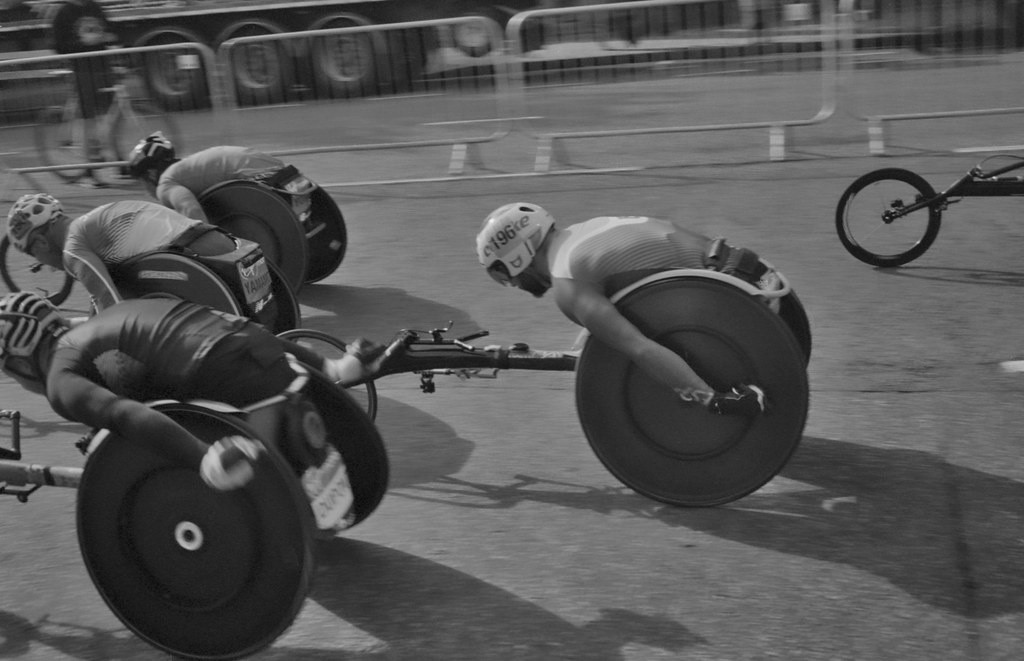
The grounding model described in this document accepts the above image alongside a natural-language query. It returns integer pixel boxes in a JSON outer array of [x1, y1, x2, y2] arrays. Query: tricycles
[[0, 175, 348, 306], [0, 364, 388, 661], [86, 231, 300, 347], [277, 258, 812, 508], [836, 155, 1024, 266]]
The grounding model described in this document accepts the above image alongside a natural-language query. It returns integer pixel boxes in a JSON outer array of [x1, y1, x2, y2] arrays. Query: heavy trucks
[[0, 0, 432, 120]]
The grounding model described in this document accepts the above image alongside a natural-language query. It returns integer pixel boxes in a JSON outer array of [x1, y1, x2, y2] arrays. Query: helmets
[[0, 290, 64, 370], [129, 129, 175, 175], [476, 202, 556, 278], [8, 193, 64, 251]]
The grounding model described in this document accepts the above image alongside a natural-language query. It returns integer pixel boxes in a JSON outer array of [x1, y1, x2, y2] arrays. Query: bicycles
[[31, 44, 181, 186]]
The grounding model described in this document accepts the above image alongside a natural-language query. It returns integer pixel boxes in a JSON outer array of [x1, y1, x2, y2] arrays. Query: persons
[[127, 131, 285, 224], [6, 192, 237, 317], [0, 291, 389, 497], [475, 203, 812, 417]]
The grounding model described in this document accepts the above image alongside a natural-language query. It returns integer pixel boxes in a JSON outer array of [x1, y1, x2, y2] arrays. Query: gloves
[[707, 391, 761, 420], [200, 443, 252, 492], [322, 355, 360, 385]]
